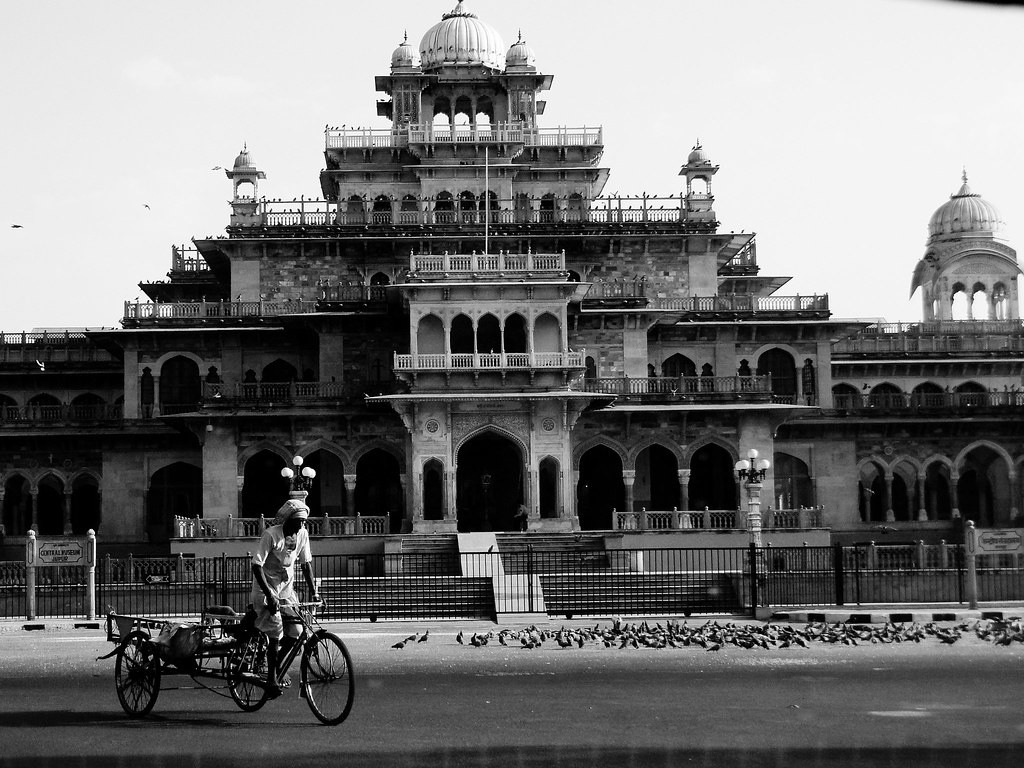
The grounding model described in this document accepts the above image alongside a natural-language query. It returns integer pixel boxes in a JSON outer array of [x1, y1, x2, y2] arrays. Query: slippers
[[283, 672, 292, 688]]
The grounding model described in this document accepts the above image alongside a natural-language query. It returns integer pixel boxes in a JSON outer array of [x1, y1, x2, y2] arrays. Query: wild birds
[[389, 630, 431, 650], [456, 614, 1024, 653], [211, 166, 222, 170], [10, 223, 24, 229], [142, 204, 151, 212], [33, 44, 1024, 420]]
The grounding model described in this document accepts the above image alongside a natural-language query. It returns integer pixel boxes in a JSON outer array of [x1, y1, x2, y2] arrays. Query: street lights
[[282, 456, 316, 506], [736, 449, 770, 572]]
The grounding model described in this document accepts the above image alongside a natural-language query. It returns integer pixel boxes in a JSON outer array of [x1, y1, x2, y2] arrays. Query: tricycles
[[106, 605, 355, 729]]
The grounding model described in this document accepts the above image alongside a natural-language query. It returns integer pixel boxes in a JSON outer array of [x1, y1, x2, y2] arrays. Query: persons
[[252, 499, 327, 697], [514, 501, 528, 531]]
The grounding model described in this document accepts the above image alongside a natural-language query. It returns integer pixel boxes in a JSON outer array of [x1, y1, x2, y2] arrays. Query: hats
[[276, 499, 310, 526]]
